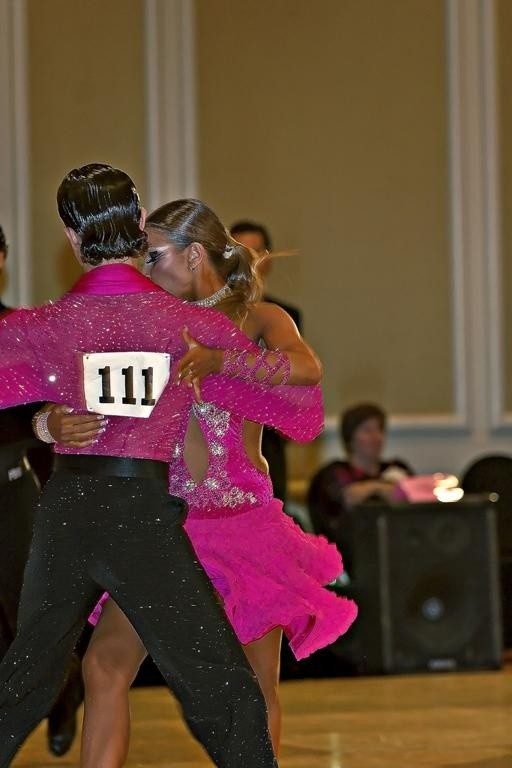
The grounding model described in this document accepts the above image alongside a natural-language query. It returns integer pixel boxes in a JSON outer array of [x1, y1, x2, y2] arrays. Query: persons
[[232, 218, 304, 503], [1, 163, 324, 768], [2, 231, 84, 756], [310, 398, 415, 514], [33, 200, 359, 768]]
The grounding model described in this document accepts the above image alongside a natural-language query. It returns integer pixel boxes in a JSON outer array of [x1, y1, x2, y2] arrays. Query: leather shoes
[[47, 715, 77, 756]]
